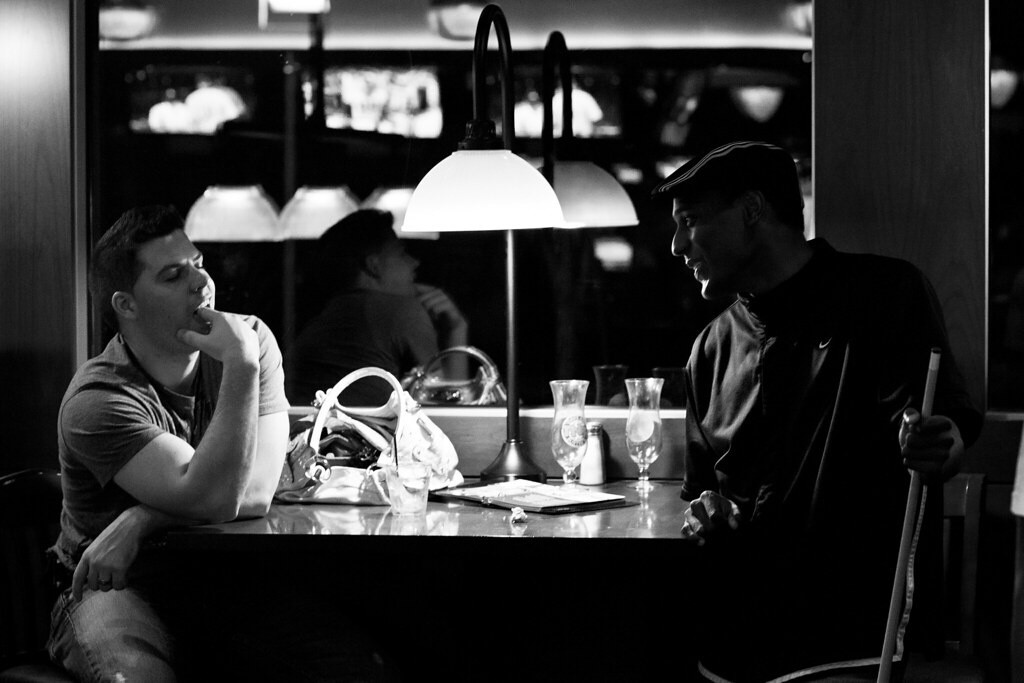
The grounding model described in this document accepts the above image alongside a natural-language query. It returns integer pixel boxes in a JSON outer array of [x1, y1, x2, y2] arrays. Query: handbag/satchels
[[403, 344, 508, 408], [276, 366, 465, 506]]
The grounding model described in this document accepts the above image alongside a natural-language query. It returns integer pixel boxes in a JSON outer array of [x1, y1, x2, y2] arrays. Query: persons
[[42, 209, 290, 683], [297, 211, 468, 406], [660, 143, 981, 683]]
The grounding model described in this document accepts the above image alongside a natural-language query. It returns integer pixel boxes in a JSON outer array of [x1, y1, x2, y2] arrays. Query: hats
[[651, 141, 803, 209]]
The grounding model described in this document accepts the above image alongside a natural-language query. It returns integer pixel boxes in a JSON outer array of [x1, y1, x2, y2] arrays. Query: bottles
[[579, 422, 606, 486]]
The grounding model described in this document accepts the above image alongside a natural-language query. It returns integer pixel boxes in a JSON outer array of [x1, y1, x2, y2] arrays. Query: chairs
[[0, 463, 86, 683], [904, 467, 986, 682]]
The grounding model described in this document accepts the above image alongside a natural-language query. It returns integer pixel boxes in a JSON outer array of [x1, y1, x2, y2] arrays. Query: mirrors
[[68, 5, 823, 425]]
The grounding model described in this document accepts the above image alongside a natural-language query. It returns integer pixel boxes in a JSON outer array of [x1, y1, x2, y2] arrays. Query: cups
[[385, 462, 432, 517]]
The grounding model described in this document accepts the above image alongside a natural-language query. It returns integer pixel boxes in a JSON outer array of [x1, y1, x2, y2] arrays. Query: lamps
[[400, 4, 567, 482], [177, 176, 277, 244], [538, 28, 642, 229], [372, 176, 434, 240], [271, 11, 363, 249]]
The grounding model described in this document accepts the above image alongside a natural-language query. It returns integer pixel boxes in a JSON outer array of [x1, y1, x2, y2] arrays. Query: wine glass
[[549, 380, 590, 490], [624, 377, 664, 490]]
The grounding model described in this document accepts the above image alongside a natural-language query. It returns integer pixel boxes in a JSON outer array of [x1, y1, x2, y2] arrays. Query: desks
[[200, 470, 756, 683]]
